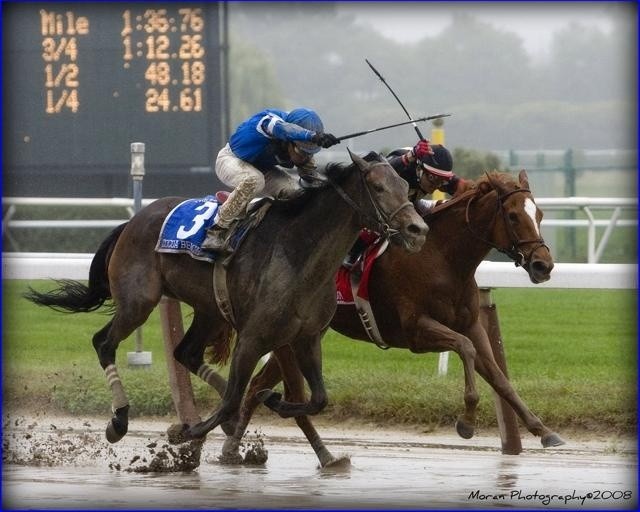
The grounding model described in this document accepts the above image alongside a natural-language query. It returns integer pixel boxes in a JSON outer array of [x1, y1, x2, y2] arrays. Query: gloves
[[312, 130, 341, 149]]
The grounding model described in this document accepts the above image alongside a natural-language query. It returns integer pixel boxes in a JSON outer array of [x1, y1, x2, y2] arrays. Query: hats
[[417, 143, 455, 178]]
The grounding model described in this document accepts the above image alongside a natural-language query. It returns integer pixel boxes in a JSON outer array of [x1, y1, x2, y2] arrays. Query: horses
[[25, 145, 430, 443], [221, 168, 565, 469]]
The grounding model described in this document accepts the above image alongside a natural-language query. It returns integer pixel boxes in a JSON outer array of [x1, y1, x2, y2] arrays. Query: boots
[[200, 223, 228, 250], [341, 237, 369, 270]]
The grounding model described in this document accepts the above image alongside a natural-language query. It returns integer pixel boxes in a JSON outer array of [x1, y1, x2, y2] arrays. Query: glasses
[[294, 146, 318, 160], [428, 174, 449, 187]]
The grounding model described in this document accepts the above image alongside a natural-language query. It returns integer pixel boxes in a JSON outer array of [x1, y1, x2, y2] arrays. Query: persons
[[200, 107, 340, 253], [342, 138, 466, 273]]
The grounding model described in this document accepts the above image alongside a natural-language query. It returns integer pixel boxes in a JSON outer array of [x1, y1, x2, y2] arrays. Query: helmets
[[287, 108, 325, 154]]
